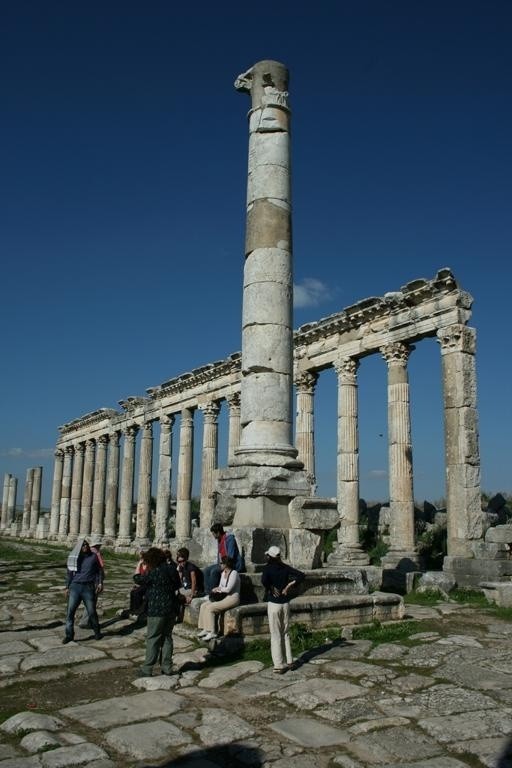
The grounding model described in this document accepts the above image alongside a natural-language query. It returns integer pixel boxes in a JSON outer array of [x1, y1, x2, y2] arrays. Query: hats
[[90, 537, 102, 547], [265, 546, 281, 558]]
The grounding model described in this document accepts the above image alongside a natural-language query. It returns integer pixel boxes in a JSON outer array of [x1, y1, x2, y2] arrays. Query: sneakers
[[197, 629, 217, 642], [95, 632, 102, 641], [63, 637, 74, 644]]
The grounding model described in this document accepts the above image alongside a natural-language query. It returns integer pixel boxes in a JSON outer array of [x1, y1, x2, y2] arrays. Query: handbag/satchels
[[209, 592, 227, 602]]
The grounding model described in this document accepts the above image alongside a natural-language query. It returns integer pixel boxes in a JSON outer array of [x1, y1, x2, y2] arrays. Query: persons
[[261, 545, 305, 673], [134, 548, 180, 676], [78, 537, 104, 629], [197, 556, 241, 642], [202, 523, 244, 600], [128, 547, 197, 622], [61, 539, 104, 644]]
